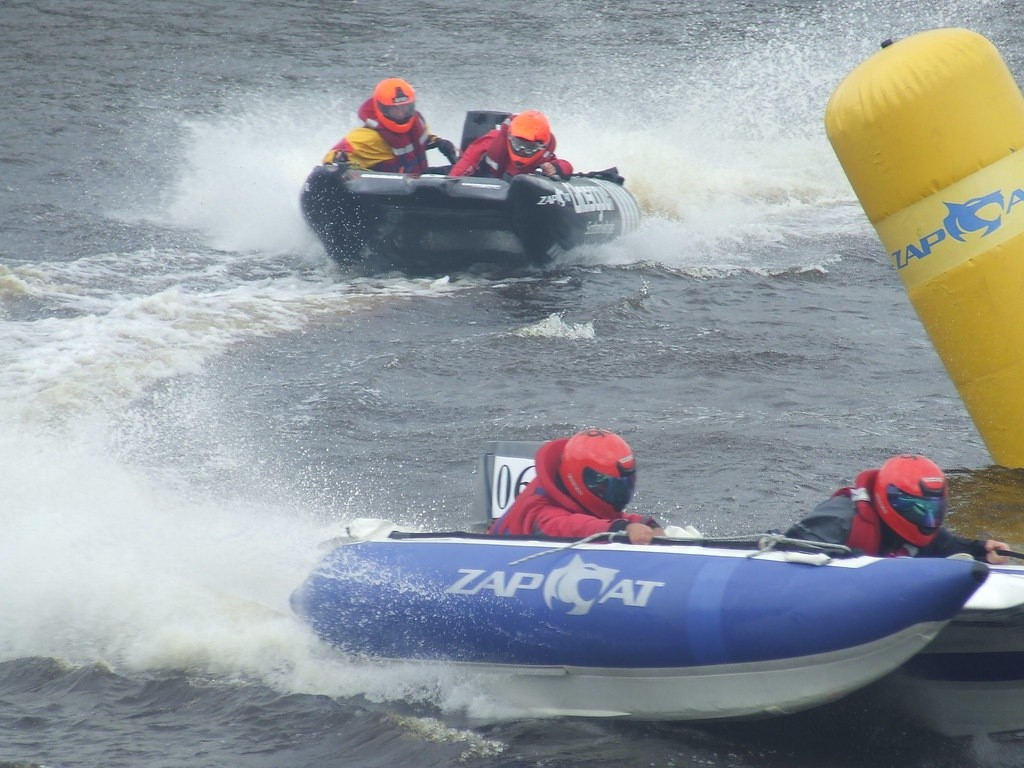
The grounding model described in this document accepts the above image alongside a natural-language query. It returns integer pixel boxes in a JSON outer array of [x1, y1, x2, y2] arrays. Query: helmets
[[874, 454, 947, 547], [559, 429, 636, 520], [374, 78, 416, 134], [509, 110, 550, 169]]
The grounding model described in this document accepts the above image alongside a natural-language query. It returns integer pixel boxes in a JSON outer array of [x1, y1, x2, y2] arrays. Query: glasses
[[510, 135, 544, 155]]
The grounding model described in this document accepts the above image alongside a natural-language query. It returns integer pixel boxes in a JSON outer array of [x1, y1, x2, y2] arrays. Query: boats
[[294, 109, 643, 261], [283, 527, 992, 720]]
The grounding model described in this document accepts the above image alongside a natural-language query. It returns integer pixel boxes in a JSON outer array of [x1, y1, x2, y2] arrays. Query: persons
[[483, 428, 665, 546], [446, 110, 573, 181], [772, 454, 1011, 565], [323, 78, 455, 175]]
